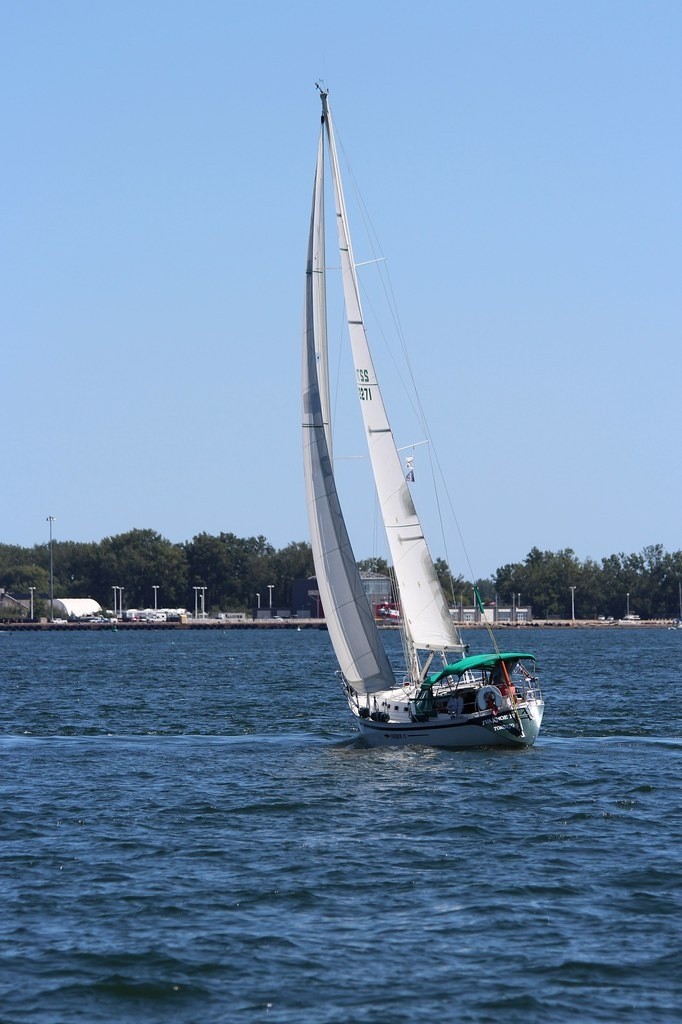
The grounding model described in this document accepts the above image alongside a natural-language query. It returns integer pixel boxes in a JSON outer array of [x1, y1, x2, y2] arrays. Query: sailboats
[[300, 82, 545, 750]]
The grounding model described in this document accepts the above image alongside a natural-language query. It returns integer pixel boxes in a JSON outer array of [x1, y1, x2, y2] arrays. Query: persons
[[112, 621, 116, 628], [489, 657, 536, 698], [447, 691, 464, 714], [377, 605, 387, 616], [385, 607, 391, 616]]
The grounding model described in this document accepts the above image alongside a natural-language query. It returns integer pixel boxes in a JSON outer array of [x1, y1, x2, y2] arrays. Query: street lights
[[111, 586, 124, 614], [256, 593, 261, 609], [266, 585, 275, 608], [28, 586, 36, 620], [569, 585, 577, 620], [626, 593, 630, 615], [152, 586, 160, 608], [46, 516, 56, 622], [193, 586, 207, 619]]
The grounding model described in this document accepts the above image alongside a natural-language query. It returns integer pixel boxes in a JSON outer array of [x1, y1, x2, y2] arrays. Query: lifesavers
[[477, 685, 503, 710]]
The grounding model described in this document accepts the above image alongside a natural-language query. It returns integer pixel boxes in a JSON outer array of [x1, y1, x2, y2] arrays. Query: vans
[[147, 613, 166, 622]]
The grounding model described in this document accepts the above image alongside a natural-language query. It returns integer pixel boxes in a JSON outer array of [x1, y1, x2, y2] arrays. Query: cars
[[598, 615, 605, 621], [50, 617, 68, 624], [131, 615, 147, 622], [607, 616, 614, 621]]
